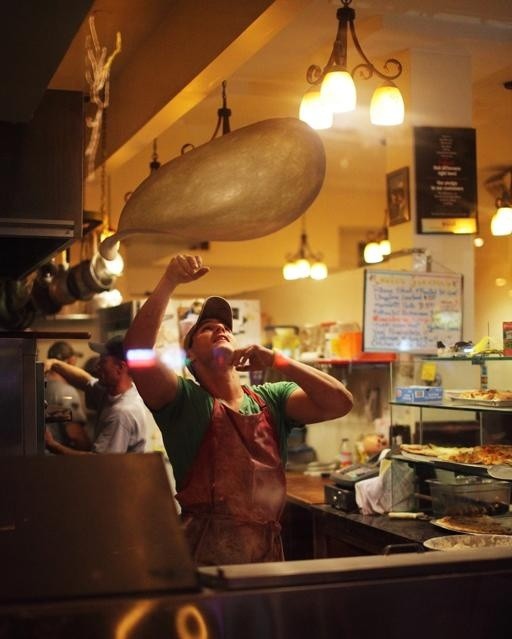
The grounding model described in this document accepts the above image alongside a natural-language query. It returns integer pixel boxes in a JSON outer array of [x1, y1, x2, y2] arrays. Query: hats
[[183, 296, 233, 351], [48, 341, 84, 361], [87, 334, 126, 360]]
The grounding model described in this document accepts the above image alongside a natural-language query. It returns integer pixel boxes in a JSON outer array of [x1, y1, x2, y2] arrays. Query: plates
[[421, 533, 512, 552]]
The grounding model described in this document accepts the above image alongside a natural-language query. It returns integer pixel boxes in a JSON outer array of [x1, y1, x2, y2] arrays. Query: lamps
[[491, 181, 512, 238], [283, 216, 328, 283], [296, 0, 405, 137]]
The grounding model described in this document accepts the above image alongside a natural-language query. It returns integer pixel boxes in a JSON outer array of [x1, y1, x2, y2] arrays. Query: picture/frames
[[386, 166, 410, 226]]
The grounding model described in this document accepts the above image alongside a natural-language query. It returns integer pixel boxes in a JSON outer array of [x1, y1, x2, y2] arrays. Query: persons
[[122, 254, 354, 565], [43, 335, 183, 520]]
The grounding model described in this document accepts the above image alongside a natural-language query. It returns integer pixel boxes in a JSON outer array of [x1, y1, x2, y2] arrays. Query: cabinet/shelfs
[[389, 353, 512, 481]]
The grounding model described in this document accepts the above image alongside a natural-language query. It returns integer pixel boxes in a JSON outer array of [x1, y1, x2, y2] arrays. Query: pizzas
[[437, 514, 511, 534], [448, 443, 512, 467]]
